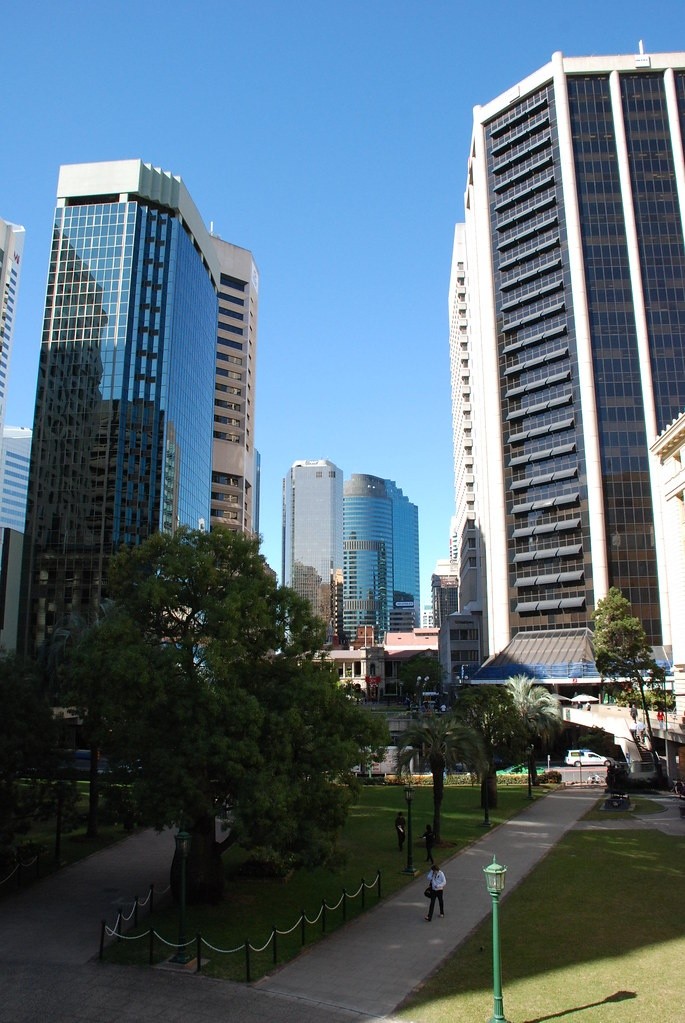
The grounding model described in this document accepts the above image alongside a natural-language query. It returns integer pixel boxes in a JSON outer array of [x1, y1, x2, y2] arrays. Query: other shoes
[[425, 917, 431, 921], [440, 914, 444, 918]]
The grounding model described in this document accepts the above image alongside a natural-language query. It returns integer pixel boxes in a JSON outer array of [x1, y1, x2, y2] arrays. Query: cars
[[565, 750, 611, 767], [497, 764, 545, 774]]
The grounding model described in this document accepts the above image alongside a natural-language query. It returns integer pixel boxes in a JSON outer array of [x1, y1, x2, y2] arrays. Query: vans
[[411, 692, 457, 710]]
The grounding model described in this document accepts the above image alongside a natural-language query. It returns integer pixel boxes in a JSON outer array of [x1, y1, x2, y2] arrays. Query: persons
[[440, 703, 446, 712], [629, 704, 638, 723], [656, 711, 664, 729], [418, 824, 434, 865], [674, 780, 683, 798], [585, 701, 590, 711], [394, 812, 406, 853], [424, 865, 446, 921]]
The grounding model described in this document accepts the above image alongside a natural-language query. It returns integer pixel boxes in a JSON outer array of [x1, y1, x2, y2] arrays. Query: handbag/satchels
[[425, 887, 432, 898]]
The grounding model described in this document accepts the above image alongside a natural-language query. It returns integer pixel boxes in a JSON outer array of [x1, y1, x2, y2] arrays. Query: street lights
[[479, 759, 493, 827], [525, 744, 535, 800], [400, 782, 419, 876], [169, 831, 197, 964], [482, 855, 508, 1023]]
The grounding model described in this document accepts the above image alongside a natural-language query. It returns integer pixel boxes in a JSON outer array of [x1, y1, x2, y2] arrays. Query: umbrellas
[[570, 693, 599, 704], [550, 693, 570, 701]]
